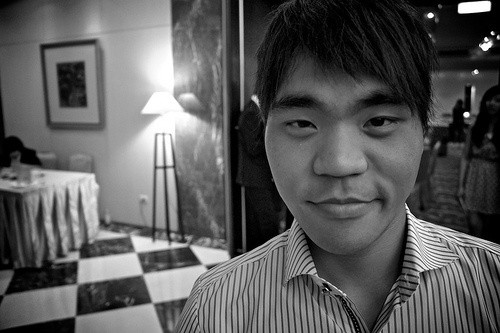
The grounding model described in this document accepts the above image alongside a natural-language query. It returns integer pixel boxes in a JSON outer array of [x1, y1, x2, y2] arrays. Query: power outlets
[[139, 195, 148, 204]]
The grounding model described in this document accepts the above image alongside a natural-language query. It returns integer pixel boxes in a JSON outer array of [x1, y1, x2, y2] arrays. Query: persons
[[174, 0, 500, 333], [446, 98, 466, 142], [462, 86, 500, 245], [0, 136, 42, 170], [234, 90, 282, 252]]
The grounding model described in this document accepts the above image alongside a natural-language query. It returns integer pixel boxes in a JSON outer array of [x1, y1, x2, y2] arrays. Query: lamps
[[139, 90, 188, 243]]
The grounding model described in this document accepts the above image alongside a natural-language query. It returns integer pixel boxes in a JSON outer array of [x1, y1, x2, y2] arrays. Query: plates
[[11, 180, 36, 186]]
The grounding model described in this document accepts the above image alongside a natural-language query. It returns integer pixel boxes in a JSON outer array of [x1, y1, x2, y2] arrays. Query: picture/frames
[[39, 37, 107, 131]]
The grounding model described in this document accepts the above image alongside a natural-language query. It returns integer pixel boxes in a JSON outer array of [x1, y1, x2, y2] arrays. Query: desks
[[0, 167, 100, 268]]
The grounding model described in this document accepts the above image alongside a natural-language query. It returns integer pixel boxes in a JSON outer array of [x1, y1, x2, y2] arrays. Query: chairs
[[68, 152, 92, 173], [35, 152, 56, 170]]
